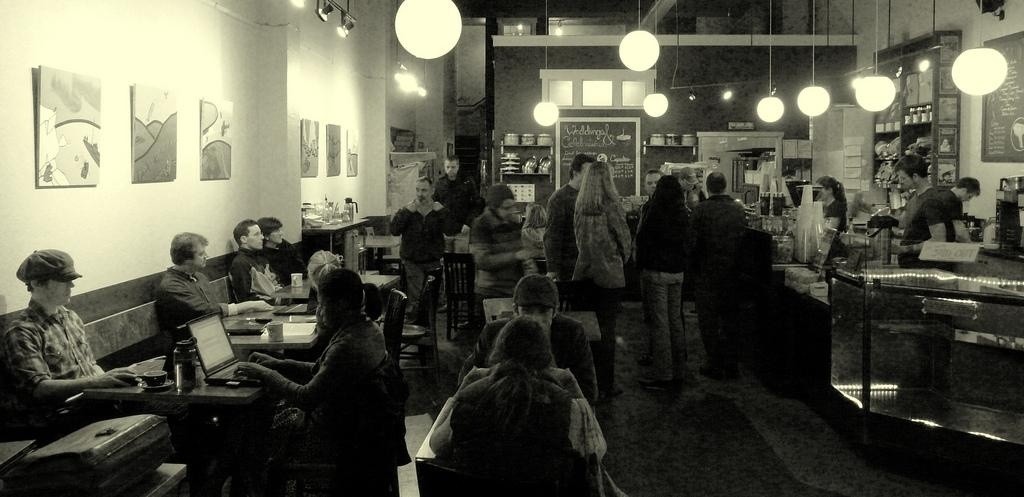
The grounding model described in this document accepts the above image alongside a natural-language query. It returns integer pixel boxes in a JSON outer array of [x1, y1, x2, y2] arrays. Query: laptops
[[225, 317, 272, 336], [185, 311, 263, 385], [120, 323, 186, 384], [272, 286, 313, 315]]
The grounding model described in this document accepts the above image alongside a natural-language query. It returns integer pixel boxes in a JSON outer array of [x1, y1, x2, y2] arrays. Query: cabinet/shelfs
[[871, 29, 962, 191], [783, 139, 811, 158], [812, 106, 873, 190], [500, 141, 554, 183], [826, 260, 1024, 496], [697, 130, 783, 200], [642, 141, 697, 155]]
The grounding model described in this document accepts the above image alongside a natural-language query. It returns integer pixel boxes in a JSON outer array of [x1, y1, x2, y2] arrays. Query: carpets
[[395, 299, 949, 497]]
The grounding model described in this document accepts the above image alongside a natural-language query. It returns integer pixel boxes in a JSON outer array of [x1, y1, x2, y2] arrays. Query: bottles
[[875, 119, 885, 132], [536, 132, 553, 147], [894, 119, 901, 130], [759, 192, 771, 218], [912, 110, 918, 123], [504, 132, 520, 146], [884, 120, 894, 131], [904, 112, 911, 124], [649, 132, 665, 145], [916, 106, 923, 122], [521, 132, 535, 147], [773, 194, 785, 218], [681, 133, 697, 146], [344, 199, 359, 223], [921, 109, 927, 121], [665, 132, 680, 146], [172, 339, 197, 392], [925, 104, 931, 121], [909, 106, 915, 124]]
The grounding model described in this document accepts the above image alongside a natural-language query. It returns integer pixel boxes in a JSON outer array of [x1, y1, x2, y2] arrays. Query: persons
[[257, 216, 307, 279], [543, 154, 591, 310], [237, 268, 411, 495], [637, 175, 693, 390], [472, 182, 543, 313], [230, 219, 284, 299], [0, 249, 137, 443], [156, 231, 273, 348], [428, 321, 625, 497], [815, 176, 851, 263], [304, 250, 346, 293], [390, 175, 459, 329], [681, 167, 699, 204], [432, 156, 473, 253], [459, 274, 597, 411], [684, 169, 748, 379], [897, 156, 946, 269], [643, 167, 664, 197], [940, 176, 980, 243], [575, 160, 633, 399], [519, 203, 548, 252]]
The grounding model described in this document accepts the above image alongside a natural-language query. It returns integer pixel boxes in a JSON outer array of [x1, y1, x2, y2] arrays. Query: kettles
[[344, 198, 358, 221]]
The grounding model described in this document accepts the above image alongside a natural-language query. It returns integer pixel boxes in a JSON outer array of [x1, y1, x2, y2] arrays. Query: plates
[[138, 380, 174, 389], [501, 153, 519, 173]]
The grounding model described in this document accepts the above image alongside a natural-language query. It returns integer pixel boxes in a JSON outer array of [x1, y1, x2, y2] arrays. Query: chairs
[[406, 265, 445, 357], [283, 462, 337, 497], [444, 252, 483, 340], [383, 255, 401, 273], [366, 227, 378, 257], [554, 280, 579, 310], [384, 288, 407, 361], [400, 274, 436, 380]]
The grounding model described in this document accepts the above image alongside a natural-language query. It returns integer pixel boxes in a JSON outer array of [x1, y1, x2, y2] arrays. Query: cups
[[137, 372, 167, 386], [291, 274, 302, 287], [268, 323, 283, 341]]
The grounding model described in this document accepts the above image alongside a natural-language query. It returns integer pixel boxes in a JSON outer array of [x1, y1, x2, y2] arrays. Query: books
[[181, 313, 236, 375], [274, 281, 312, 319]]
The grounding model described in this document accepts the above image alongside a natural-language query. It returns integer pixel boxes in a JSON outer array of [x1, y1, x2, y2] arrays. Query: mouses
[[120, 377, 141, 383]]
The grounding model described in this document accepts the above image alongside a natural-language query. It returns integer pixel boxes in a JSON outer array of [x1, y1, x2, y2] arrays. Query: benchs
[[84, 276, 237, 360]]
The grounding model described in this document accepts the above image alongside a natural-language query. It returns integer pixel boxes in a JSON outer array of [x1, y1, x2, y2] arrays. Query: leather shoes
[[721, 367, 740, 379], [699, 366, 720, 379]]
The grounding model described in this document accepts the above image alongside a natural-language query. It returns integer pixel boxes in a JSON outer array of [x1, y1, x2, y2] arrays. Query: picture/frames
[[981, 31, 1024, 161], [728, 120, 755, 130], [301, 119, 319, 176], [347, 153, 357, 176], [326, 124, 341, 176], [447, 142, 453, 158], [201, 100, 232, 179], [37, 65, 101, 186], [131, 83, 176, 182]]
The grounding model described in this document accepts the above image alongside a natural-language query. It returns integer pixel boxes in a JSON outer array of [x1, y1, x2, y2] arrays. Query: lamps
[[643, 0, 669, 117], [393, 0, 463, 59], [723, 86, 732, 98], [315, 0, 334, 21], [798, 0, 830, 117], [534, 0, 559, 125], [336, 13, 354, 36], [977, 0, 1006, 19], [619, 0, 660, 70], [689, 89, 697, 100], [952, 0, 1008, 96], [756, 0, 784, 122], [856, 0, 896, 111], [769, 82, 777, 94]]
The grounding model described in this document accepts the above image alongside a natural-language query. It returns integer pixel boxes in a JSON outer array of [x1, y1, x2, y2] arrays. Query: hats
[[485, 185, 514, 209], [513, 274, 559, 308], [679, 167, 699, 185], [17, 249, 83, 284]]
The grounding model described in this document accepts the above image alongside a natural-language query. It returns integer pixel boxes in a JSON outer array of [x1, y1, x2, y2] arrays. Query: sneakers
[[638, 377, 673, 392], [599, 386, 623, 401], [675, 373, 688, 388]]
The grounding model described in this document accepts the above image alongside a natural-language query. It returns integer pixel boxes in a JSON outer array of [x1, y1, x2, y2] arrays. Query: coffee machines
[[993, 176, 1024, 252]]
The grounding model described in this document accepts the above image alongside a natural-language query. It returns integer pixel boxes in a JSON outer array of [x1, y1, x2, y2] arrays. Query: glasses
[[318, 302, 330, 308], [248, 232, 263, 237], [899, 175, 910, 180], [969, 193, 972, 200]]
